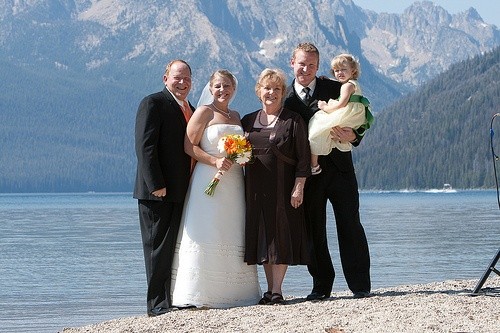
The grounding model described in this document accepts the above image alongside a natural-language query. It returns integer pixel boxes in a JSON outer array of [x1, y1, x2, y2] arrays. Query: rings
[[339, 136, 341, 139], [299, 201, 301, 203]]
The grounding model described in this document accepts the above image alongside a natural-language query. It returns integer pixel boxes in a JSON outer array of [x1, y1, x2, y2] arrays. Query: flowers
[[203, 135, 252, 197]]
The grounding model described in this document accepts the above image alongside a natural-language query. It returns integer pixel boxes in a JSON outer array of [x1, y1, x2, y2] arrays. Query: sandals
[[311, 165, 322, 175]]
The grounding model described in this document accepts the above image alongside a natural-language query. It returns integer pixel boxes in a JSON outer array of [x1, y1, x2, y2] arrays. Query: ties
[[182, 100, 197, 177], [302, 87, 312, 106]]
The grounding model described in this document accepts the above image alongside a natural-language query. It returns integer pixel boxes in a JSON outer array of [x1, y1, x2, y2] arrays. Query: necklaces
[[211, 102, 230, 118], [259, 107, 282, 126]]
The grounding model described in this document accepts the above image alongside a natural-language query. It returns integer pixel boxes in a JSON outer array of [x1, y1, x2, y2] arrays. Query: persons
[[134, 59, 312, 318], [308, 54, 374, 176], [281, 42, 371, 301]]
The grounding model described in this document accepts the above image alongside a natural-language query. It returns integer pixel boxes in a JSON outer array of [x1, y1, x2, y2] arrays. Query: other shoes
[[307, 289, 331, 301], [149, 308, 174, 316], [258, 291, 285, 305], [354, 290, 370, 298]]
